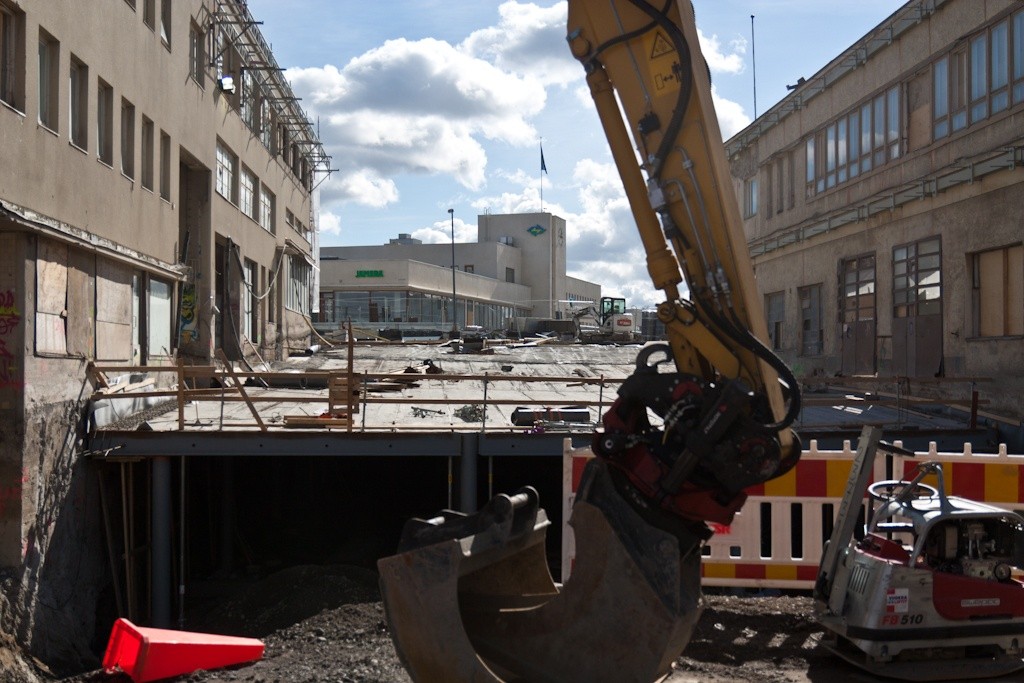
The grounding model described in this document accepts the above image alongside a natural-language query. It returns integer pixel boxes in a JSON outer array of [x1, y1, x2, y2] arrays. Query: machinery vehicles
[[574, 296, 646, 345], [375, 1, 803, 683]]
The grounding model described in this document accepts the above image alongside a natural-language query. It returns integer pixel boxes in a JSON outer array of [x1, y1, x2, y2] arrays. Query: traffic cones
[[101, 618, 266, 683]]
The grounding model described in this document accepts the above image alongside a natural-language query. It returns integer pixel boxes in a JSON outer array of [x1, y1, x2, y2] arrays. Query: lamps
[[218, 77, 236, 95]]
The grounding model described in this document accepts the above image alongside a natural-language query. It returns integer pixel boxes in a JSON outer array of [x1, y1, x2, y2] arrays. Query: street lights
[[448, 209, 456, 338]]
